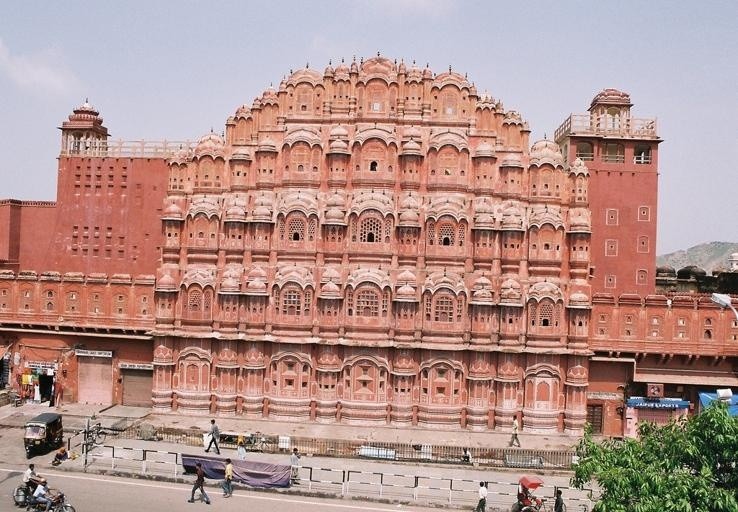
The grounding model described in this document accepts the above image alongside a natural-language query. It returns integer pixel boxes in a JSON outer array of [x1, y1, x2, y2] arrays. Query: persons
[[474, 481, 487, 512], [237, 434, 246, 460], [290, 448, 302, 484], [22, 464, 43, 491], [204, 418, 220, 455], [51, 447, 67, 466], [220, 457, 233, 498], [461, 446, 472, 462], [553, 490, 563, 512], [33, 478, 61, 512], [187, 462, 210, 504], [508, 414, 520, 447]]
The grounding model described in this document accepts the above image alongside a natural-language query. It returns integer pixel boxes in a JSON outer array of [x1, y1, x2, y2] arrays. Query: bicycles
[[12, 485, 49, 507], [87, 422, 107, 446], [242, 431, 277, 454]]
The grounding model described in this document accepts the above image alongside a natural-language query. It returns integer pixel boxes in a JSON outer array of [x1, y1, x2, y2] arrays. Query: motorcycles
[[26, 488, 79, 512], [21, 413, 65, 460]]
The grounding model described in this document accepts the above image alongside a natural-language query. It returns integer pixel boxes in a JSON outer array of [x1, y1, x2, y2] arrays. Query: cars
[[218, 432, 265, 450]]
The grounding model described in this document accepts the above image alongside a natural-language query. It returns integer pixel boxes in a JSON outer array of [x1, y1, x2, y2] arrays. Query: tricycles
[[509, 477, 565, 512]]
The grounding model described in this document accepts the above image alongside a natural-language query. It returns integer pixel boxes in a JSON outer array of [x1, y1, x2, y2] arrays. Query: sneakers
[[188, 500, 194, 502], [216, 451, 219, 454], [205, 449, 209, 452], [205, 500, 210, 503]]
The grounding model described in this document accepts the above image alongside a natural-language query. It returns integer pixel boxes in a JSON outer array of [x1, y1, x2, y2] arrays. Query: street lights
[[707, 292, 737, 326]]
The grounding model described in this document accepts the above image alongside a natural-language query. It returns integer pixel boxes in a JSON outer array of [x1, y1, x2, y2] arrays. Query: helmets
[[40, 478, 47, 485]]
